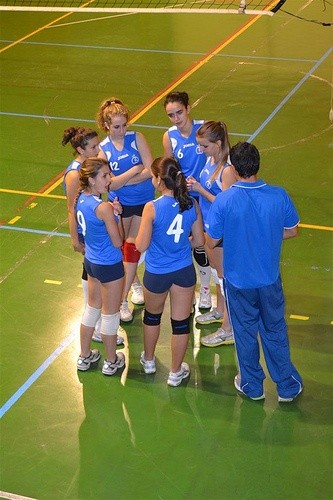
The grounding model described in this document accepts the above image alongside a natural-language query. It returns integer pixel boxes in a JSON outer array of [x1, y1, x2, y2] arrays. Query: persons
[[134, 155, 206, 387], [61, 96, 154, 376], [161, 92, 303, 403]]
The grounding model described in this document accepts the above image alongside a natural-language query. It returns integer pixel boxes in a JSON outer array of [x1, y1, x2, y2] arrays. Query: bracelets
[[118, 214, 120, 216]]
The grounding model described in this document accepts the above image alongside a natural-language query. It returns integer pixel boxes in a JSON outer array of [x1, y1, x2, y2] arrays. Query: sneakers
[[139, 350, 156, 374], [130, 283, 145, 304], [201, 328, 234, 347], [120, 299, 133, 322], [167, 361, 190, 387], [92, 330, 124, 345], [77, 349, 100, 371], [195, 307, 224, 325], [102, 352, 125, 375], [190, 297, 196, 313], [234, 374, 265, 401], [277, 385, 303, 402], [199, 286, 212, 308]]
